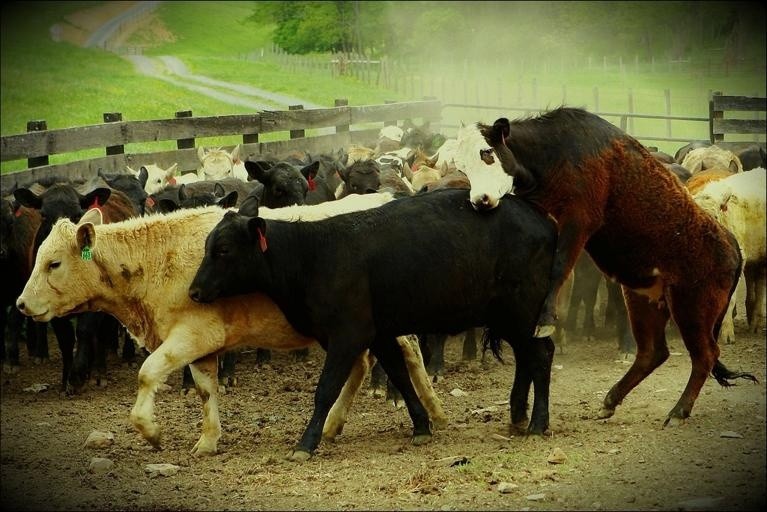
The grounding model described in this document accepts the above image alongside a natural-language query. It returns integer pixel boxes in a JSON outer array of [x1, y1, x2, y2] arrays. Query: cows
[[1, 103, 767, 464]]
